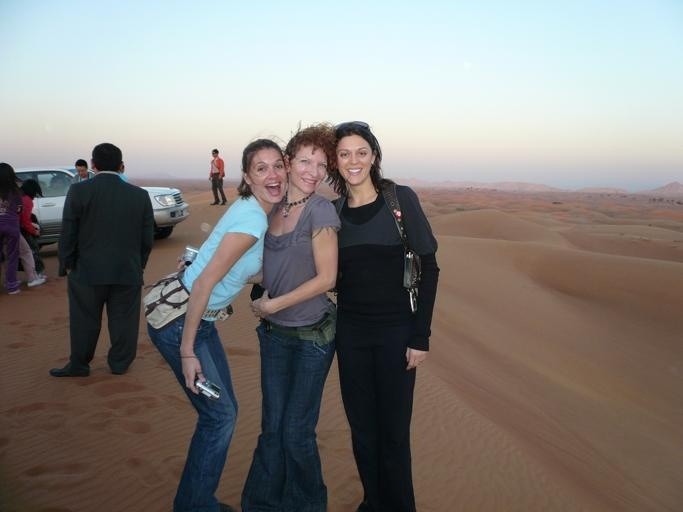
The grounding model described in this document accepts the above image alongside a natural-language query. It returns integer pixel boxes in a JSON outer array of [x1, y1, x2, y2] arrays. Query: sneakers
[[27, 278, 46, 288]]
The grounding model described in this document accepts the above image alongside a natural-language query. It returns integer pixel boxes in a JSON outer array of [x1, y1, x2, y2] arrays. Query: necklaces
[[282, 191, 315, 218]]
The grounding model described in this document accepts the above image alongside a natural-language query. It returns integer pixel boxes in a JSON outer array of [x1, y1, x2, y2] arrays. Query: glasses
[[333, 120, 370, 131]]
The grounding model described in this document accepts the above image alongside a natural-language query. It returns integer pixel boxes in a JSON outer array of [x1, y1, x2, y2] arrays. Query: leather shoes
[[49, 364, 79, 377]]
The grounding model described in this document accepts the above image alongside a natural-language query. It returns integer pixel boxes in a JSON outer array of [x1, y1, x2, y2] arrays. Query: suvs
[[14, 166, 190, 253]]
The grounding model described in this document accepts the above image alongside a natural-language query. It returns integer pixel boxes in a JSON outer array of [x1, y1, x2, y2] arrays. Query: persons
[[324, 120, 440, 511], [120, 162, 128, 182], [240, 125, 342, 511], [48, 145, 155, 377], [0, 162, 48, 295], [208, 148, 227, 206], [69, 159, 95, 186], [142, 137, 288, 512]]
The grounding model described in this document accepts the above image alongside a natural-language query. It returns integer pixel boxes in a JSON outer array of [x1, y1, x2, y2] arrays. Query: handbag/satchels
[[379, 184, 421, 314], [142, 275, 190, 334]]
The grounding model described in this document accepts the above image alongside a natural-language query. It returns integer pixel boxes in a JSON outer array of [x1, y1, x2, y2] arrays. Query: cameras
[[196, 377, 221, 401], [182, 246, 198, 267]]
